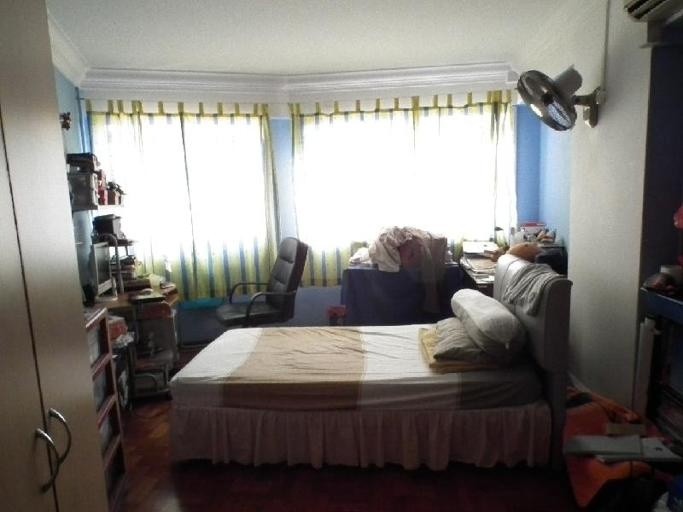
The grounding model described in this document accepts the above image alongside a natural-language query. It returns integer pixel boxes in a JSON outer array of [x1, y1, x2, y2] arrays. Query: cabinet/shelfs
[[1, 0, 107, 512], [75, 304, 126, 491]]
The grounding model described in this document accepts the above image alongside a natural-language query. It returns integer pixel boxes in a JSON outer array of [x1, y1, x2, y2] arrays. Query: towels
[[502, 262, 573, 317]]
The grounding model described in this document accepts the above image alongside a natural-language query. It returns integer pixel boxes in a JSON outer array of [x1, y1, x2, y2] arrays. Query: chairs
[[214, 237, 308, 331]]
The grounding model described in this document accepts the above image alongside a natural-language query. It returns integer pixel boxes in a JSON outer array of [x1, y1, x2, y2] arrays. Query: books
[[567, 432, 644, 457], [595, 436, 683, 466]]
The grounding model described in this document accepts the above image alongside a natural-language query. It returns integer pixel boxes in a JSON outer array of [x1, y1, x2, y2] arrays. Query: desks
[[458, 252, 498, 299], [90, 230, 180, 397], [629, 286, 683, 449], [340, 246, 461, 325]]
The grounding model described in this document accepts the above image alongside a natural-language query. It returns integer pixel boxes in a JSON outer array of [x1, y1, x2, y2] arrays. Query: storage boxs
[[91, 216, 120, 238]]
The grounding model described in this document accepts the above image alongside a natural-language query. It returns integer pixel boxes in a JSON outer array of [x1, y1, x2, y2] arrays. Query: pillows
[[432, 316, 502, 363], [449, 287, 526, 356]]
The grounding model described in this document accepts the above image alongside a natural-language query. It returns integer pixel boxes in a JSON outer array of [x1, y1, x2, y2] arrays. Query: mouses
[[139, 288, 154, 294]]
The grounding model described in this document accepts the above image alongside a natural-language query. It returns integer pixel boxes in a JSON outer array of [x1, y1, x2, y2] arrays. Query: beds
[[165, 252, 574, 470]]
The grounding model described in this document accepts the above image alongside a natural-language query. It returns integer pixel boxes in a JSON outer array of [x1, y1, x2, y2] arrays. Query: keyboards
[[128, 289, 165, 303]]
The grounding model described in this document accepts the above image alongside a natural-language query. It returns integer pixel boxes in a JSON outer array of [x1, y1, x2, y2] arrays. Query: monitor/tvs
[[87, 238, 117, 299]]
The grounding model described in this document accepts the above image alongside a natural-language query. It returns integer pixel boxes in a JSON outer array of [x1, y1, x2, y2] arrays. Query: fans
[[517, 65, 602, 131]]
